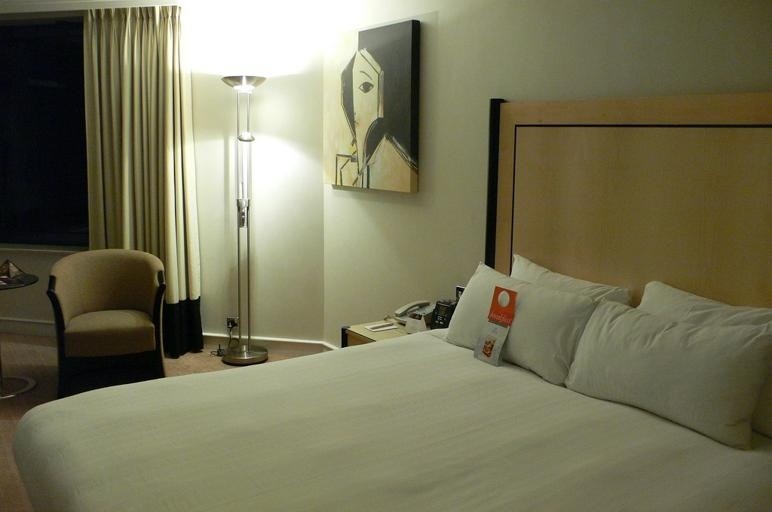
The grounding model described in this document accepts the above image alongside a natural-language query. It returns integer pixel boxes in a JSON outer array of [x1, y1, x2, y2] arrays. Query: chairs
[[46, 248, 168, 396]]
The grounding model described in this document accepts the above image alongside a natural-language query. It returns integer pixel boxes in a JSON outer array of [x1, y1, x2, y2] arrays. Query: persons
[[335, 48, 417, 194]]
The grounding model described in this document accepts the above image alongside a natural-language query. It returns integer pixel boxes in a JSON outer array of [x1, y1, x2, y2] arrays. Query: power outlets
[[226, 317, 239, 329]]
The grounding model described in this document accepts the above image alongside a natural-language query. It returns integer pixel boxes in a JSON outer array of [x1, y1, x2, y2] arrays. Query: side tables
[[0, 257, 40, 401]]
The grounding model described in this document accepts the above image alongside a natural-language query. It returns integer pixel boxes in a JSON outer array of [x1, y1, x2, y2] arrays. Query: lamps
[[219, 75, 269, 364]]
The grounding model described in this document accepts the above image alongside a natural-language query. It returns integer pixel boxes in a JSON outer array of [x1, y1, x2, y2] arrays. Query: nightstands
[[342, 305, 439, 347]]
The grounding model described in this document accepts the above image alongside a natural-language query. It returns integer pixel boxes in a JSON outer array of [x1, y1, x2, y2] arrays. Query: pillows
[[639, 282, 772, 442], [508, 252, 630, 325], [450, 260, 583, 386], [568, 298, 772, 447]]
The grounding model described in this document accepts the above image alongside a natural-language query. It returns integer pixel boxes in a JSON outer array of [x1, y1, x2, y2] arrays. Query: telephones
[[384, 299, 437, 326]]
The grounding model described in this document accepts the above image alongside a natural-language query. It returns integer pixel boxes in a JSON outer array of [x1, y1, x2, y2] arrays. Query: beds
[[14, 96, 772, 512]]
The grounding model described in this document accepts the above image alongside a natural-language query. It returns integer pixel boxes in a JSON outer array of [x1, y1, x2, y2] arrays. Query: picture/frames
[[321, 20, 420, 194]]
